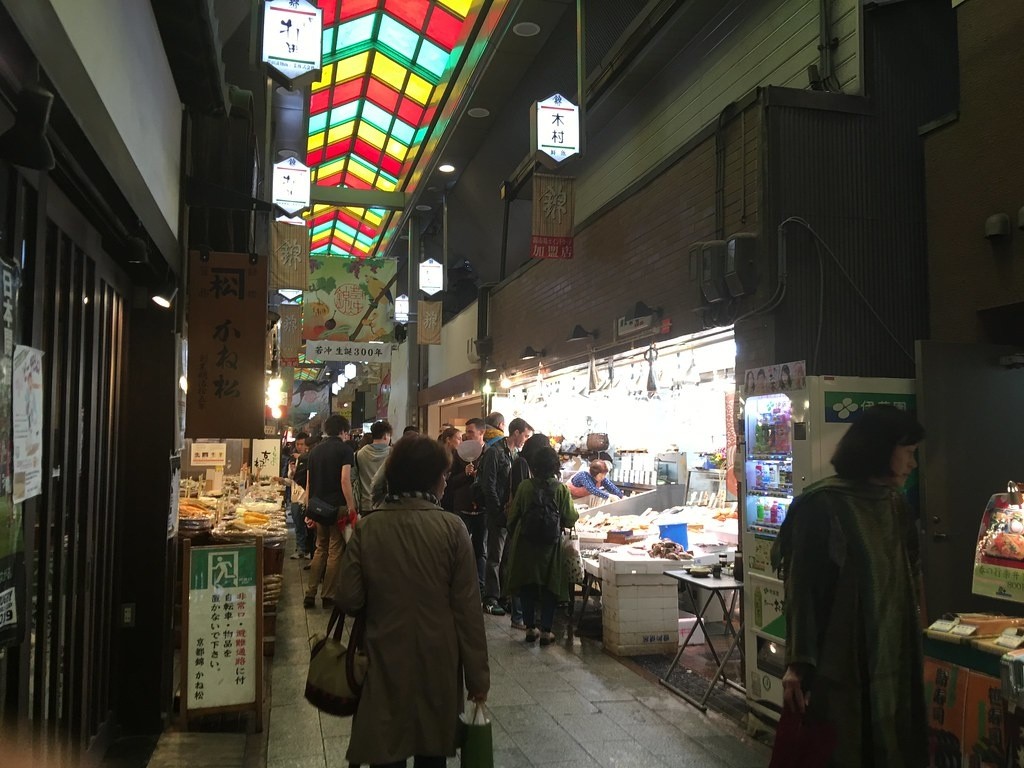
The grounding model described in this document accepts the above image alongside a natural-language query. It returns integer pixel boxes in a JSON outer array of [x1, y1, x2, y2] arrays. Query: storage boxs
[[678, 610, 705, 645]]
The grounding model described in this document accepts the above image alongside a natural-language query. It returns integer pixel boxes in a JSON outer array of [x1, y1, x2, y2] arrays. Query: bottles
[[754, 461, 793, 493], [755, 499, 790, 529], [735, 551, 743, 580], [754, 406, 792, 455]]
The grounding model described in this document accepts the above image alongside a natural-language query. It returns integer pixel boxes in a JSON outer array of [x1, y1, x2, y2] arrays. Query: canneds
[[718, 555, 727, 567]]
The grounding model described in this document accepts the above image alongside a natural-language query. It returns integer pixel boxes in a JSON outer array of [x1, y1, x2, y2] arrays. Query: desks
[[208, 503, 287, 657], [574, 556, 603, 643], [659, 568, 744, 712]]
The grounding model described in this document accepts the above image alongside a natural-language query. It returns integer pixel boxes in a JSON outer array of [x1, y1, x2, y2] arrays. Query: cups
[[710, 563, 722, 578], [719, 553, 727, 573]]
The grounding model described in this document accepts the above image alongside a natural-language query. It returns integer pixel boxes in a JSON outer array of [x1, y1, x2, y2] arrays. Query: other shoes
[[290, 553, 303, 559], [526, 627, 540, 642], [303, 597, 314, 608], [305, 553, 311, 559], [322, 599, 335, 609], [540, 632, 555, 645]]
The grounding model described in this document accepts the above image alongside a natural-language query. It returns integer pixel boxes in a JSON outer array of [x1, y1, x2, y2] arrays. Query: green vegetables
[[991, 544, 1016, 552]]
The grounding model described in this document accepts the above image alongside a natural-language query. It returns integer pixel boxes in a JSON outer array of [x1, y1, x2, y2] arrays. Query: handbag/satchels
[[502, 468, 514, 519], [457, 701, 494, 768], [303, 603, 362, 718], [305, 496, 339, 527], [561, 524, 585, 584]]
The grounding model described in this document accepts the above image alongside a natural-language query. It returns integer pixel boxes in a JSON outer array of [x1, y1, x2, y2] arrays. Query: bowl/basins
[[684, 567, 711, 577]]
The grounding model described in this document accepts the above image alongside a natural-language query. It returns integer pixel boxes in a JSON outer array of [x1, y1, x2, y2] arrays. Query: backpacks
[[521, 477, 563, 547]]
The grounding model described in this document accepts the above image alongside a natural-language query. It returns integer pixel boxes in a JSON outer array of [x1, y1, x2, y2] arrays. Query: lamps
[[481, 361, 504, 373], [625, 301, 658, 321], [519, 346, 548, 360], [565, 324, 599, 340]]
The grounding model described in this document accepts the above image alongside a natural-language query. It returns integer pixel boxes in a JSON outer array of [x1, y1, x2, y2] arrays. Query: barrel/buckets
[[587, 432, 609, 451], [657, 522, 689, 551]]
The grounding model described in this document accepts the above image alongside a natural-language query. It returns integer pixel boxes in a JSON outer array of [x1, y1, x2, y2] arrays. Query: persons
[[269, 413, 530, 615], [505, 443, 579, 644], [771, 401, 934, 768], [332, 433, 490, 768], [562, 458, 627, 503], [505, 435, 552, 630]]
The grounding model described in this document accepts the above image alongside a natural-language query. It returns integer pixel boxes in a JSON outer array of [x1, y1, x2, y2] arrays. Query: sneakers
[[482, 597, 505, 615], [511, 621, 526, 630]]
